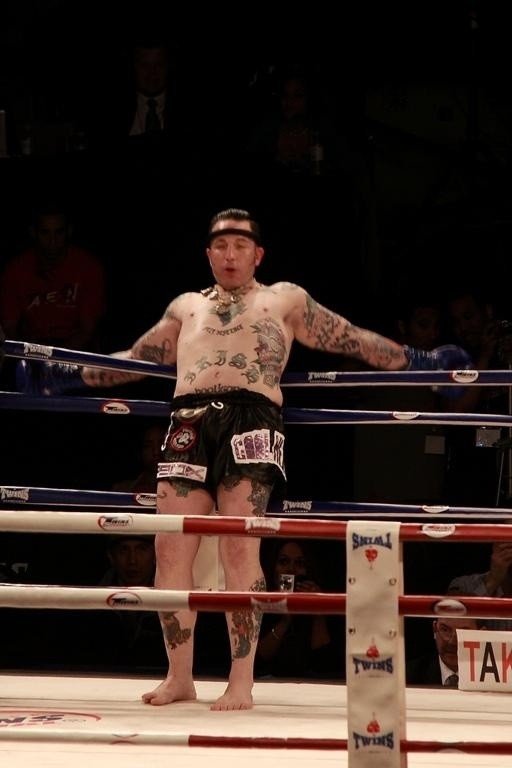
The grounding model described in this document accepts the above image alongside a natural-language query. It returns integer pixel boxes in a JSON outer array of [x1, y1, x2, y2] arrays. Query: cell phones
[[280, 573, 295, 593]]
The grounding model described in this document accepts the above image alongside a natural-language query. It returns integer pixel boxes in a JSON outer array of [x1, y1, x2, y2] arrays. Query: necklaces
[[198, 278, 258, 321]]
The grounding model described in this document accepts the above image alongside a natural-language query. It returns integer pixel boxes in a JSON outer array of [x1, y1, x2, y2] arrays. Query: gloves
[[15, 359, 90, 396], [403, 344, 477, 399]]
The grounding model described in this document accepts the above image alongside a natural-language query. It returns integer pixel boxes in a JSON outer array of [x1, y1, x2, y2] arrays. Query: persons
[[0, 24, 512, 689], [15, 209, 475, 716]]
